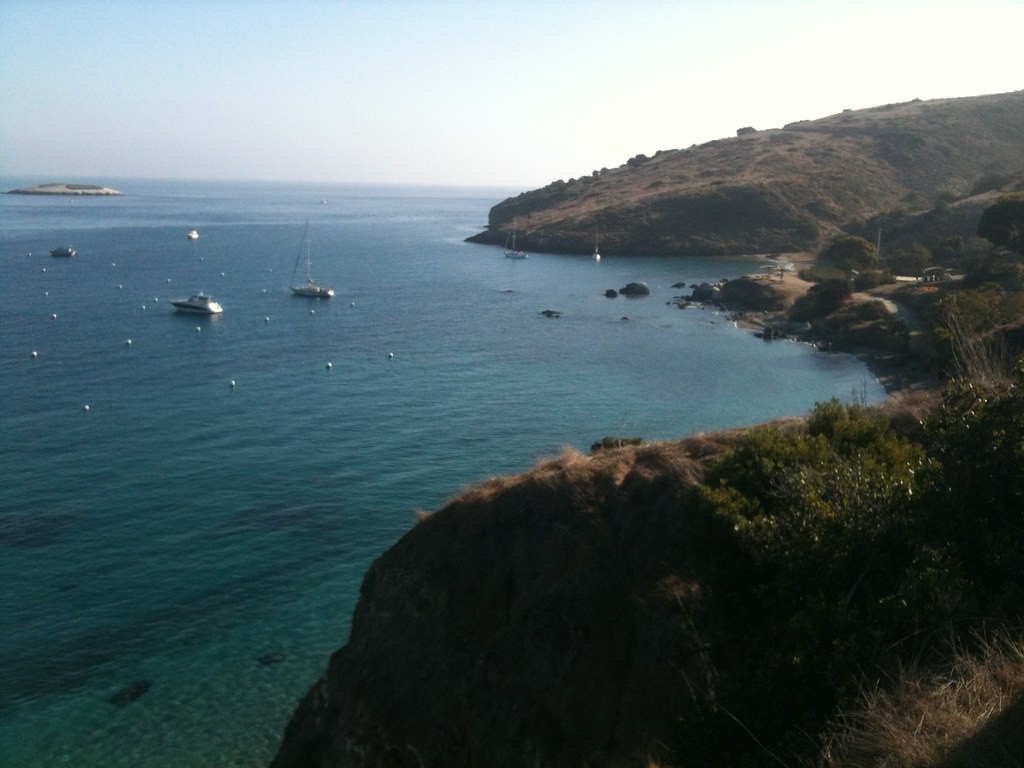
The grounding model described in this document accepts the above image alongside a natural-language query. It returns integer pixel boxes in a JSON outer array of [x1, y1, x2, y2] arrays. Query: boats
[[170, 292, 223, 314], [49, 247, 77, 258], [186, 229, 200, 239]]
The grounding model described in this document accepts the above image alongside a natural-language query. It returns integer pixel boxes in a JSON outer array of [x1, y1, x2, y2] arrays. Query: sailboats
[[593, 226, 601, 260], [287, 216, 337, 296], [502, 214, 527, 261]]
